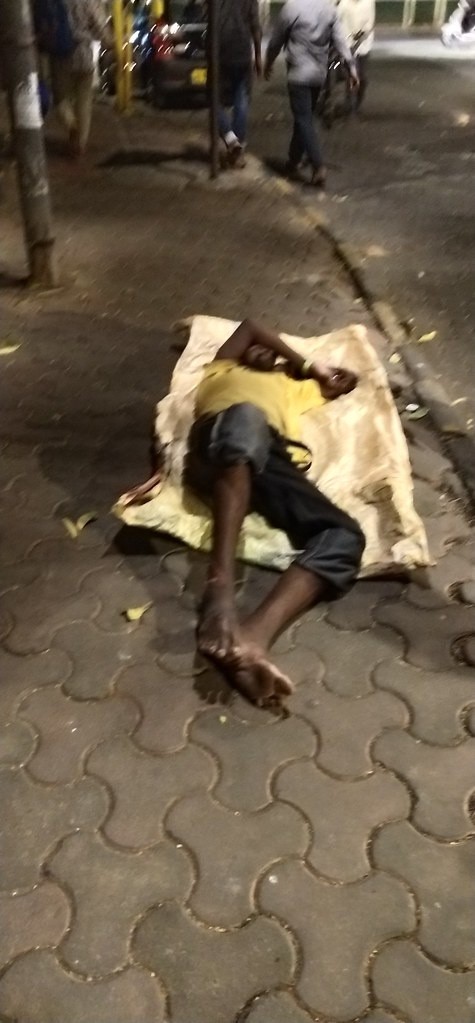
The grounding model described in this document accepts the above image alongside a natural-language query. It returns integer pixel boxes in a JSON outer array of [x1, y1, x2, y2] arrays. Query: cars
[[136, 22, 214, 107]]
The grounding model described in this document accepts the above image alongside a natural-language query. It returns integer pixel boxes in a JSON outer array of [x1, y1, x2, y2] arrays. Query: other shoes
[[225, 140, 248, 171], [65, 128, 86, 161], [282, 159, 329, 189]]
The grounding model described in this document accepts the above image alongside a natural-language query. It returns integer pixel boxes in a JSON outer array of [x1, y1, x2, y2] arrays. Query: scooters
[[439, 0, 475, 46]]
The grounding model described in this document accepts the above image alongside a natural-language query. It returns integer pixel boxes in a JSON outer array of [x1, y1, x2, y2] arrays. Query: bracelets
[[302, 359, 312, 373]]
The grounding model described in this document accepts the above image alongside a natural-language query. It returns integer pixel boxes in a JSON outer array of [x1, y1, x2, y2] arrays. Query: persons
[[207, 0, 264, 170], [31, 0, 113, 162], [338, 1, 376, 113], [184, 317, 366, 709], [263, 1, 360, 185]]
